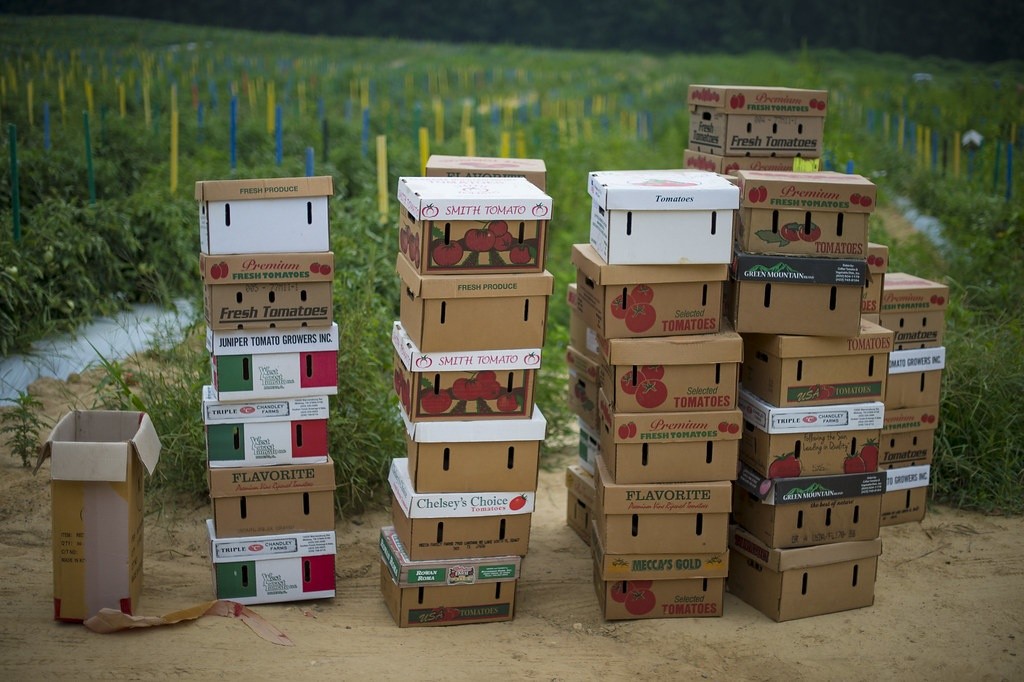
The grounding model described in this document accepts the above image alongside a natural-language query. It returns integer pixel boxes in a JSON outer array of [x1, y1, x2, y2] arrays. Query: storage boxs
[[194, 176, 334, 256], [33, 410, 162, 621], [391, 321, 542, 423], [380, 526, 522, 629], [397, 252, 555, 354], [206, 454, 334, 540], [425, 154, 547, 194], [397, 177, 554, 275], [398, 401, 547, 493], [207, 519, 336, 605], [199, 252, 334, 330], [205, 321, 339, 401], [200, 385, 329, 469], [388, 457, 536, 561], [564, 84, 950, 624]]
